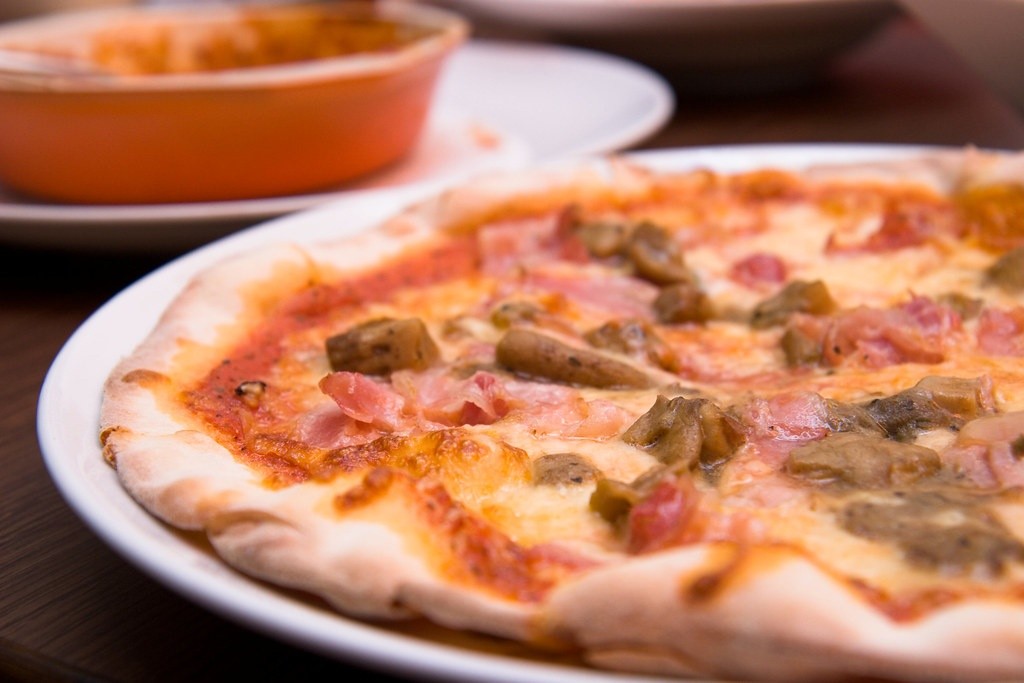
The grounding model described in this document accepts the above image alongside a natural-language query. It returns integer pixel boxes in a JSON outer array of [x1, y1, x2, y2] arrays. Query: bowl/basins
[[0, 8, 468, 200]]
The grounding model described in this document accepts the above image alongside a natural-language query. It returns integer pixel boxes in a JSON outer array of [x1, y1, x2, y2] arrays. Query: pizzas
[[100, 166, 1024, 681]]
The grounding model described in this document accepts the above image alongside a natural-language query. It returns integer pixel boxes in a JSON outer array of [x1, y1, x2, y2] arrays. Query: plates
[[36, 147, 1024, 683], [0, 39, 681, 248]]
